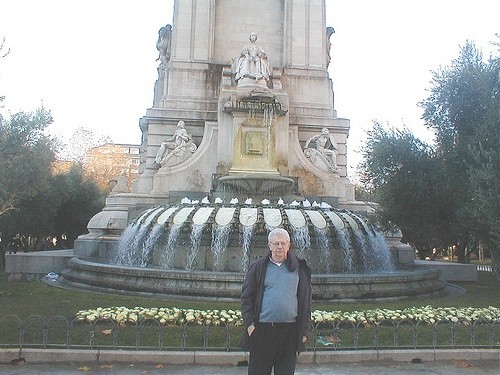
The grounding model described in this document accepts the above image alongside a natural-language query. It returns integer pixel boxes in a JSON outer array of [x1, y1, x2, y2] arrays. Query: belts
[[258, 323, 297, 327]]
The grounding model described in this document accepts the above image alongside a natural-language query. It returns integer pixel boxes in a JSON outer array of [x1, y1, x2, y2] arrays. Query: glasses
[[270, 242, 289, 246]]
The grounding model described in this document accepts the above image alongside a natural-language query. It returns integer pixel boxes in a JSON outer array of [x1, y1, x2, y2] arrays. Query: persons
[[156, 24, 172, 66], [304, 128, 342, 170], [237, 228, 312, 375], [234, 32, 271, 82], [152, 121, 189, 164], [326, 27, 335, 68]]
